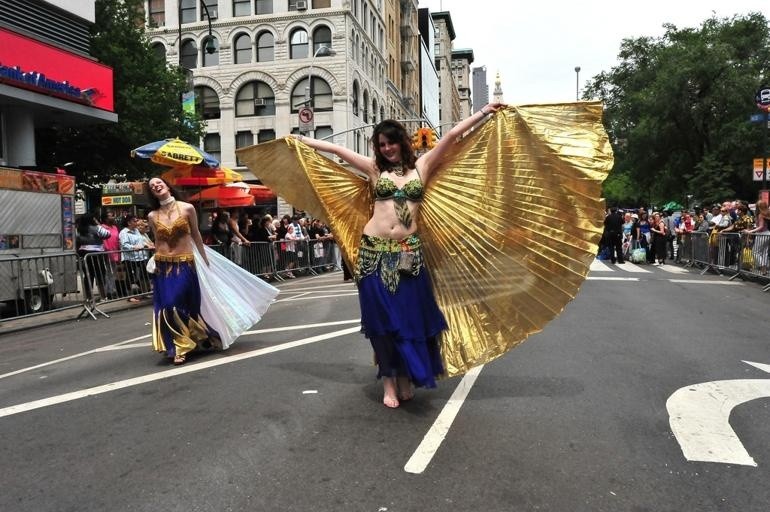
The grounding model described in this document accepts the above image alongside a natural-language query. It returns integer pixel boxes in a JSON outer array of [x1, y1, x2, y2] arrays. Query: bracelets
[[298, 134, 304, 141], [479, 107, 488, 118]]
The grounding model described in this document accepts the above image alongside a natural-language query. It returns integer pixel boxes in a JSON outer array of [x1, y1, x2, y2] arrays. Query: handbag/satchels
[[632, 240, 648, 263]]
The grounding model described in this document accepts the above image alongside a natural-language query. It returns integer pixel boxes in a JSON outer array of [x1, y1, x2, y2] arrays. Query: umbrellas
[[235, 180, 273, 198], [160, 163, 241, 200], [187, 184, 255, 206], [129, 134, 220, 171]]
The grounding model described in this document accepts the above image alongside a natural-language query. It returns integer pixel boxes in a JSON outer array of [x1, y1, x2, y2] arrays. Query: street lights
[[177, 0, 215, 112], [574, 65, 581, 101], [305, 46, 337, 138]]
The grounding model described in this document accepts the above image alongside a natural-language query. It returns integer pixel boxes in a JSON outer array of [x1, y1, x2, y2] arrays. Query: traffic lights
[[412, 127, 437, 152]]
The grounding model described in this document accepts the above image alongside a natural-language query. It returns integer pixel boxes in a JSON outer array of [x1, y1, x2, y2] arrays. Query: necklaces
[[392, 160, 409, 175], [159, 196, 175, 206]]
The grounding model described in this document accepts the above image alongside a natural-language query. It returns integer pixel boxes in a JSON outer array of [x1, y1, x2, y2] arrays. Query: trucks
[[0, 167, 77, 314]]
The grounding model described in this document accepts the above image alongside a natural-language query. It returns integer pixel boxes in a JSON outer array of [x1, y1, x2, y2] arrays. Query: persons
[[145, 176, 225, 366], [284, 100, 511, 411], [598, 198, 770, 281], [76, 205, 355, 303]]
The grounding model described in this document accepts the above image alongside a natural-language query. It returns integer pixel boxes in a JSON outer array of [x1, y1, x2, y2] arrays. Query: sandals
[[383, 377, 416, 409], [174, 354, 186, 365]]
